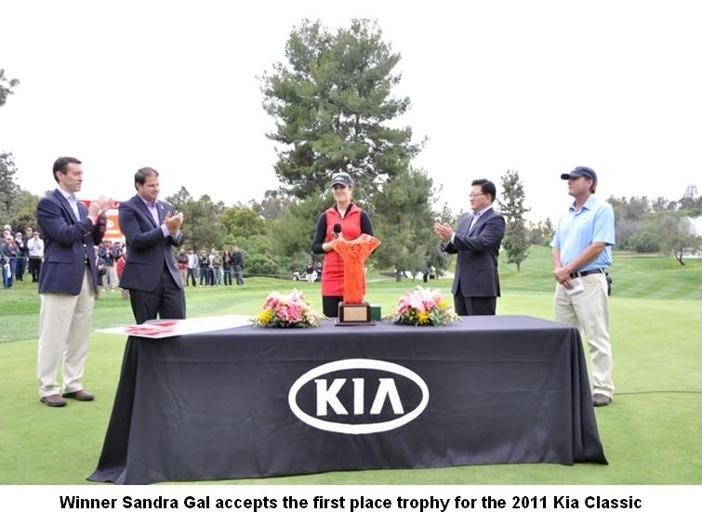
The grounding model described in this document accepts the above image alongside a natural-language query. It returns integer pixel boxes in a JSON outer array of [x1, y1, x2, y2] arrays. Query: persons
[[1, 221, 247, 289], [315, 261, 321, 276], [547, 167, 615, 407], [433, 178, 505, 316], [301, 263, 311, 278], [32, 157, 113, 407], [313, 173, 376, 317], [118, 167, 187, 323]]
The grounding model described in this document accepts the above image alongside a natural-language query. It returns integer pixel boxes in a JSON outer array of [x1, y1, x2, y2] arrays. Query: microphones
[[334, 223, 342, 238]]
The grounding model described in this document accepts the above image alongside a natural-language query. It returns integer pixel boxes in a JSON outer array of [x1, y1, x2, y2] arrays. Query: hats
[[561, 166, 597, 182], [329, 172, 353, 189]]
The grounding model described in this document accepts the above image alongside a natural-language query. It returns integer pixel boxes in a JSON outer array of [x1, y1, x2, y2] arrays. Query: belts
[[570, 268, 605, 278]]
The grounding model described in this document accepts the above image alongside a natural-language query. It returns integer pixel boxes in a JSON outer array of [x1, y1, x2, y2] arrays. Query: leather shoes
[[592, 393, 610, 406], [62, 390, 93, 401], [41, 394, 65, 406]]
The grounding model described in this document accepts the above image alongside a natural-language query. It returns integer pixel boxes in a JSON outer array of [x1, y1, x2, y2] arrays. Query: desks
[[86, 314, 608, 483]]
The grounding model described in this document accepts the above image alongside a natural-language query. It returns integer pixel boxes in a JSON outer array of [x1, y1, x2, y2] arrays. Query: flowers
[[251, 288, 320, 328], [393, 286, 464, 329]]
